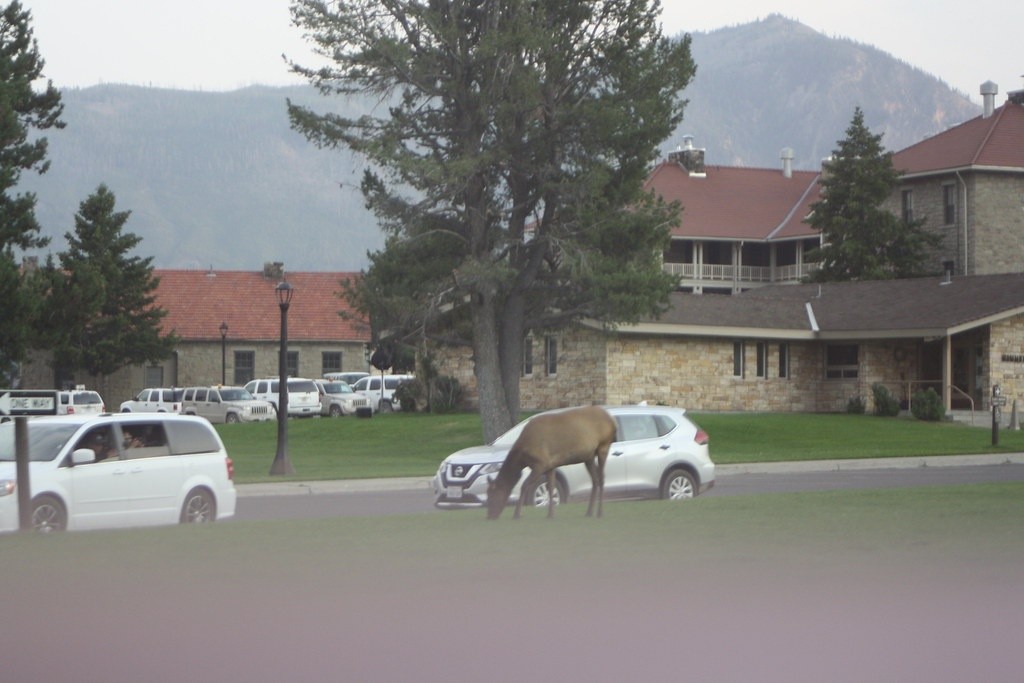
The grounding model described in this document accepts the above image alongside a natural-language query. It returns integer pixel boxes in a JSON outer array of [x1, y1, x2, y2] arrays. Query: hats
[[92, 433, 107, 444]]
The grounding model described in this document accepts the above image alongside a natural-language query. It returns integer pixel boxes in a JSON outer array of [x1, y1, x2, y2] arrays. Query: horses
[[486, 405, 617, 520]]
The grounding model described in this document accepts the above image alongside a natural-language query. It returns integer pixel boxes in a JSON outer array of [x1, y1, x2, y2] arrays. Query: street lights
[[219, 320, 228, 386], [268, 279, 297, 476]]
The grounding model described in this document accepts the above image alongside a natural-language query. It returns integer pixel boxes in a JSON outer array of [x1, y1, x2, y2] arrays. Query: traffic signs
[[0, 390, 58, 417]]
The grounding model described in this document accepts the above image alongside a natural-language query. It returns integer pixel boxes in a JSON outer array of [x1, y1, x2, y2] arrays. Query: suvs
[[180, 385, 276, 424], [431, 401, 716, 509], [56, 387, 105, 414], [120, 387, 186, 415], [312, 379, 372, 417]]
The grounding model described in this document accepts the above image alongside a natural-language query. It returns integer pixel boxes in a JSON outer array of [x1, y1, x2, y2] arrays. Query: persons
[[123, 431, 144, 450], [83, 433, 120, 462]]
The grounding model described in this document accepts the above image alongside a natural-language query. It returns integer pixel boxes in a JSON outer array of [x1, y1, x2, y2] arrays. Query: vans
[[245, 377, 322, 416], [351, 375, 417, 415], [0, 417, 237, 532], [323, 372, 369, 385]]
